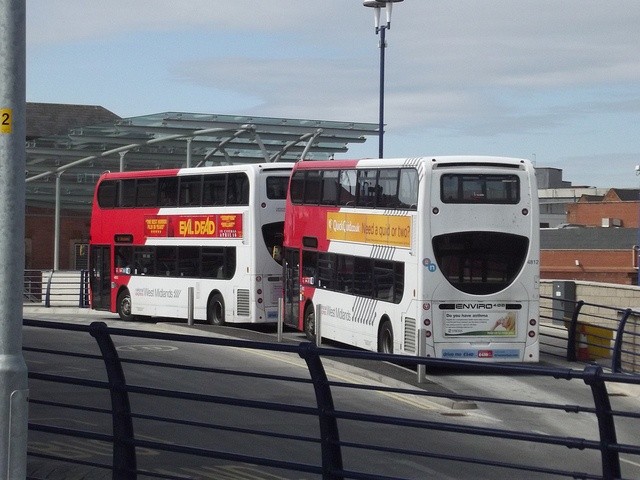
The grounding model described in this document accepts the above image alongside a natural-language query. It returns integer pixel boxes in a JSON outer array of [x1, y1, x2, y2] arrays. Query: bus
[[273, 155, 540, 367], [87, 162, 339, 326]]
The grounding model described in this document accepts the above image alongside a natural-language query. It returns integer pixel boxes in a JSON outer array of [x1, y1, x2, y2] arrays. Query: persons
[[489, 312, 515, 331]]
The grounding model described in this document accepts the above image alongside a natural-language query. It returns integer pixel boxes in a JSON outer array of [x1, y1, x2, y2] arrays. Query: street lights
[[363, 0, 405, 160]]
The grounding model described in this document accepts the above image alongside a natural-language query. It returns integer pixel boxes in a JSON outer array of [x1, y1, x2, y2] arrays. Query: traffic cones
[[577, 324, 589, 361]]
